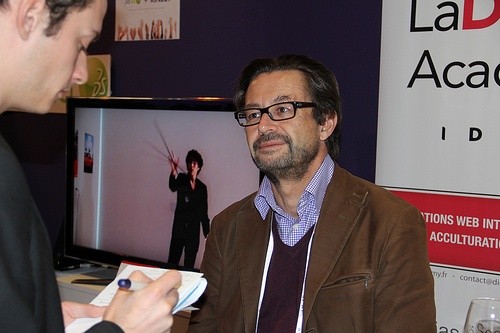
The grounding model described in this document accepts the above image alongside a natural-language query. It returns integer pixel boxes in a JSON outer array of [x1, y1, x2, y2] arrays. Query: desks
[[56, 265, 197, 333]]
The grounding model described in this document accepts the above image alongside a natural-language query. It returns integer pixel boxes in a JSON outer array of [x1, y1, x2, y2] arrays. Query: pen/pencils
[[117, 279, 148, 293]]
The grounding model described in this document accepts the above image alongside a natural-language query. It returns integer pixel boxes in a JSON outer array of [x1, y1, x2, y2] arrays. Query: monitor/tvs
[[63, 97, 267, 274]]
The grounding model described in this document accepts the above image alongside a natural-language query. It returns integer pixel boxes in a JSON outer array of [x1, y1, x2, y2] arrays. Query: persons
[[0, 0, 182, 333], [167, 149, 210, 271], [188, 55, 436, 333]]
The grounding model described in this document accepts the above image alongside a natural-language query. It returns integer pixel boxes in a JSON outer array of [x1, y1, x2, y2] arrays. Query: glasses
[[234, 102, 314, 127]]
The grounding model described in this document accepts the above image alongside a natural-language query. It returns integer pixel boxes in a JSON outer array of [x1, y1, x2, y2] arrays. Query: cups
[[464, 297, 500, 333]]
